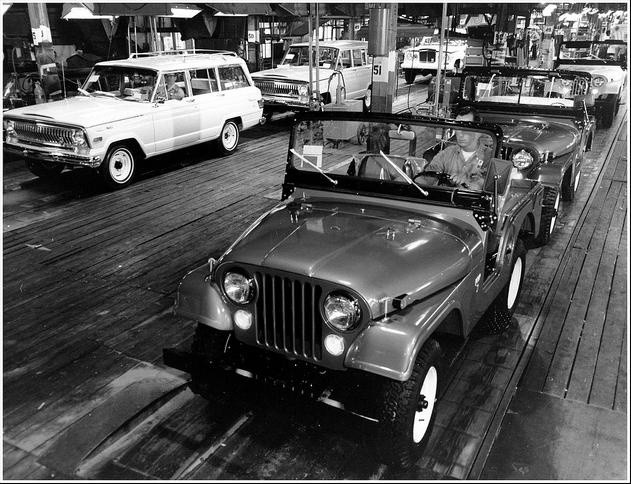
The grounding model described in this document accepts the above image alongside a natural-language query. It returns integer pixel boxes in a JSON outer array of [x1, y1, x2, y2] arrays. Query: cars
[[3, 45, 265, 186]]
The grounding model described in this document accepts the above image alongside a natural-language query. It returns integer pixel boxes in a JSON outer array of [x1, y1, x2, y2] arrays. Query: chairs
[[357, 152, 415, 185]]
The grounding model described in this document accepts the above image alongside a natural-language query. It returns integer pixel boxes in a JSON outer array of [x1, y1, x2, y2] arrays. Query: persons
[[425, 107, 492, 190], [157, 74, 184, 99]]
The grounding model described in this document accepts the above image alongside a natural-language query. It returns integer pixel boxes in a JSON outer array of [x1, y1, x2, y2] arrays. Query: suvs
[[548, 36, 627, 126], [400, 26, 487, 83], [157, 106, 554, 470], [423, 65, 597, 245], [250, 38, 380, 120]]
